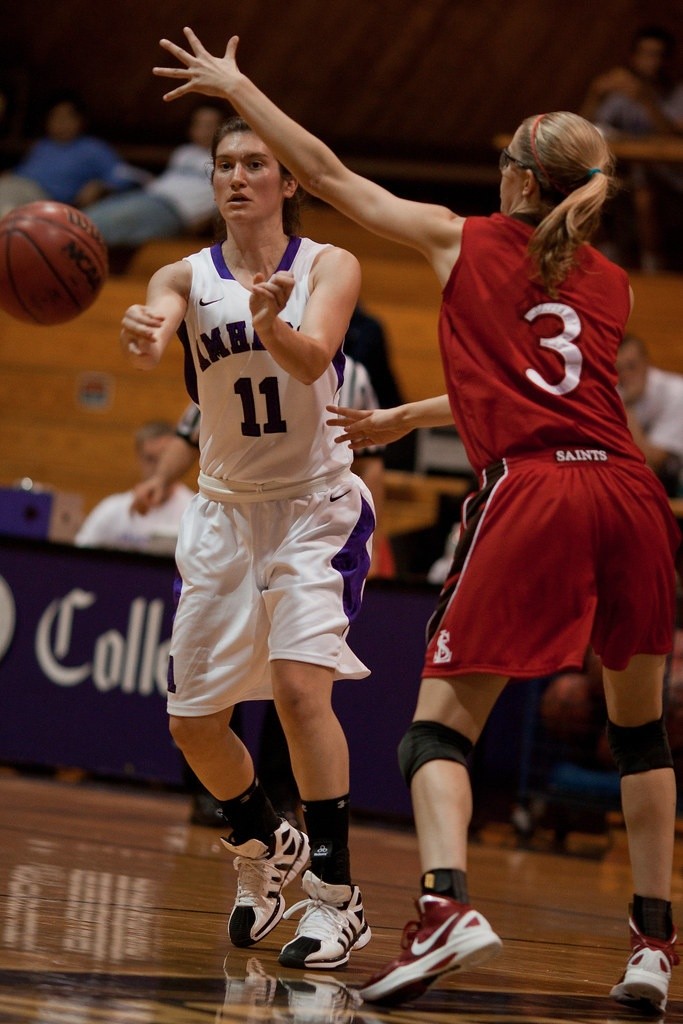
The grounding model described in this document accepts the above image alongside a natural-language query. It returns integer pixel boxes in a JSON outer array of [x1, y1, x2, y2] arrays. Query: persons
[[118, 117, 372, 968], [149, 27, 683, 1018], [78, 305, 683, 590], [581, 32, 682, 277], [0, 100, 227, 250], [609, 333, 683, 508]]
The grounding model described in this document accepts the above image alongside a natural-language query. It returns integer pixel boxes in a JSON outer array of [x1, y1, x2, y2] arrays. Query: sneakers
[[277, 869, 372, 968], [359, 893, 503, 1003], [220, 817, 311, 948], [610, 912, 680, 1016], [221, 954, 283, 1017], [279, 970, 363, 1024]]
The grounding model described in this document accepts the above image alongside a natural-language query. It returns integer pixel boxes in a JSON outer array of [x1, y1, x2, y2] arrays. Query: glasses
[[498, 147, 550, 190]]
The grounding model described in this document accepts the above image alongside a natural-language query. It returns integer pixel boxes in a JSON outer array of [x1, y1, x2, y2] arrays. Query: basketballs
[[0, 200, 110, 326]]
